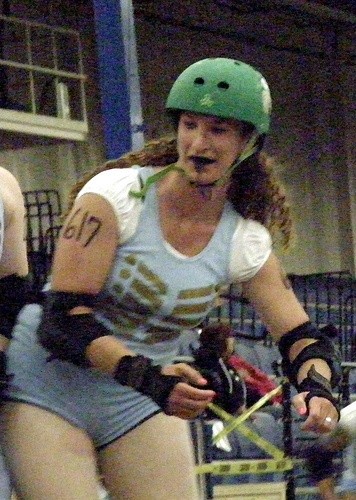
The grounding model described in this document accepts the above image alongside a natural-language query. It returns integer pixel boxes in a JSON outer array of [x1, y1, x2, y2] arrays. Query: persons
[[0, 56, 341, 500], [196, 323, 288, 414], [305, 398, 356, 500], [0, 164, 32, 499]]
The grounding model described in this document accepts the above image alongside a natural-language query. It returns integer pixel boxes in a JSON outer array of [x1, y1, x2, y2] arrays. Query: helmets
[[165, 58, 271, 134]]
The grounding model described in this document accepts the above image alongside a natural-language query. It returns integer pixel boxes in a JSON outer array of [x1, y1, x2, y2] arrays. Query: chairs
[[195, 344, 356, 484]]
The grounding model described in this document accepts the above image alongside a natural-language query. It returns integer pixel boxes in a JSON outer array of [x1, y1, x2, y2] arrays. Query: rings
[[325, 417, 334, 425]]
